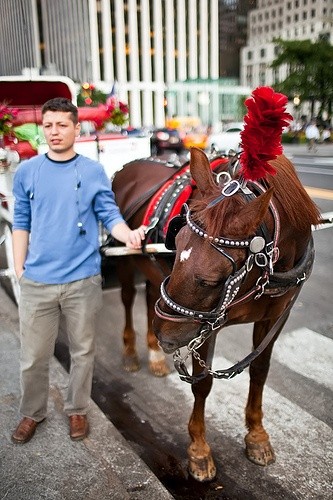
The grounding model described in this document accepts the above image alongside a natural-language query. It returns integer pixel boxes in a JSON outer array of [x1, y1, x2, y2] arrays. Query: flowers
[[77, 78, 130, 129], [0, 97, 22, 147]]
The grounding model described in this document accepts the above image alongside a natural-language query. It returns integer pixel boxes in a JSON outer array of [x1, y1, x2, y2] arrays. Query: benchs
[[7, 102, 109, 158]]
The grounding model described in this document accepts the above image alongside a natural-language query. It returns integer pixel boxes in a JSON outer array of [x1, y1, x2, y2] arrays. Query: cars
[[150, 129, 185, 157], [206, 123, 246, 159]]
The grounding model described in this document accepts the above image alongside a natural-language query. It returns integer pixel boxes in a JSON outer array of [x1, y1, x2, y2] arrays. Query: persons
[[305, 119, 320, 152], [11, 97, 147, 444]]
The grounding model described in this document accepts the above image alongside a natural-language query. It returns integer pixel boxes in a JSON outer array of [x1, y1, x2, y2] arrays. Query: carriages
[[0, 74, 316, 482]]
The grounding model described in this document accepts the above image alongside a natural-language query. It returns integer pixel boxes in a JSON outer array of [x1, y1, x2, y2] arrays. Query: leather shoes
[[70, 415, 89, 441], [11, 417, 46, 444]]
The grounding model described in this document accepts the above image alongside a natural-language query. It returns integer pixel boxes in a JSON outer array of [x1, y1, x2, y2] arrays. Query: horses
[[109, 149, 324, 485]]
